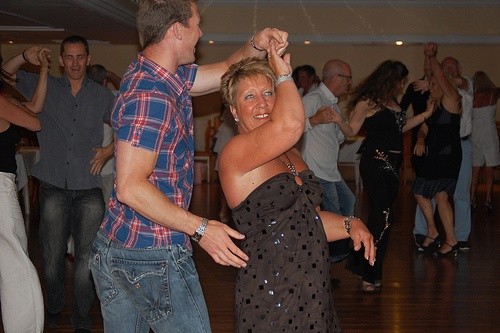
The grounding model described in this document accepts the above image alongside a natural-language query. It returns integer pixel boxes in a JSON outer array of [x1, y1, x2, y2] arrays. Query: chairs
[[14, 149, 39, 237], [194, 119, 219, 184]]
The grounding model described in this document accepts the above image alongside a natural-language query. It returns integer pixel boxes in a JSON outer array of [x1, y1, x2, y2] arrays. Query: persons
[[0, 35, 122, 333], [292, 38, 471, 293], [471, 71, 500, 208], [217, 36, 376, 333], [87, 1, 289, 333], [495, 96, 500, 127]]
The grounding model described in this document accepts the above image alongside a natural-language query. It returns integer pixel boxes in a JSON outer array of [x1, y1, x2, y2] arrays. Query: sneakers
[[47, 308, 61, 325], [76, 328, 91, 333]]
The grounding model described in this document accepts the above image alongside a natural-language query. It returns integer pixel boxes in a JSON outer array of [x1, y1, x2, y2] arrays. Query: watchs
[[192, 218, 208, 242]]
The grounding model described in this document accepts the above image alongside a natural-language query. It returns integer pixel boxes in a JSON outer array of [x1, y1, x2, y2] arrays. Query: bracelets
[[23, 50, 27, 61], [422, 113, 426, 120], [250, 34, 264, 51], [275, 74, 292, 88], [343, 216, 360, 234]]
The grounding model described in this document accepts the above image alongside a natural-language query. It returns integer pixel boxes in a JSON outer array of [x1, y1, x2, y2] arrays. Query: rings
[[268, 53, 271, 57]]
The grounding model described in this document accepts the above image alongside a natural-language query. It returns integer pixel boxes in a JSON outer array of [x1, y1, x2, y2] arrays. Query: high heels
[[483, 202, 495, 212], [469, 199, 478, 213]]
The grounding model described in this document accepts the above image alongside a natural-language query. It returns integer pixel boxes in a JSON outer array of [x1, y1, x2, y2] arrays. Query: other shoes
[[415, 233, 470, 259], [359, 280, 377, 294]]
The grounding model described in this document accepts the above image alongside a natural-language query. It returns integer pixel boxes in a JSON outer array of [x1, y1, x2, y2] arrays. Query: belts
[[460, 134, 471, 141]]
